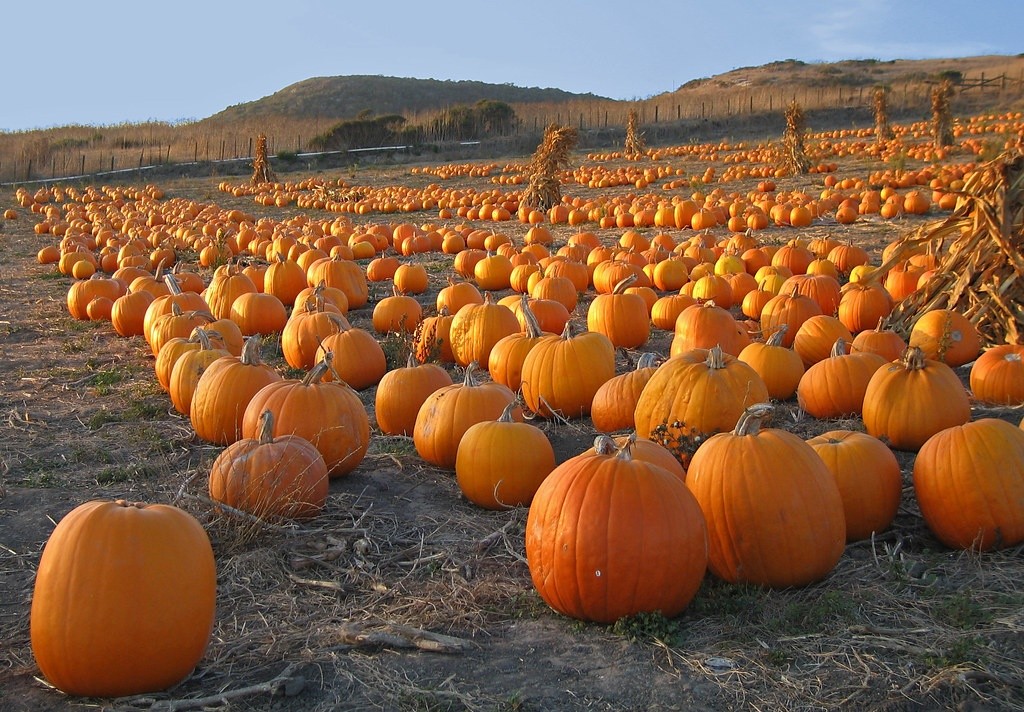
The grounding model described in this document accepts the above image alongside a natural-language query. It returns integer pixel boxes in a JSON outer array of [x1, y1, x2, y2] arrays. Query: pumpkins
[[211, 112, 1024, 232], [4, 183, 1024, 622], [29, 498, 217, 698]]
